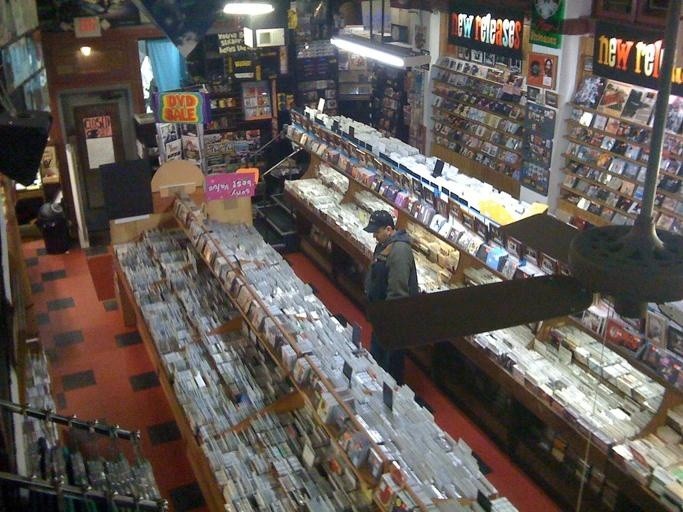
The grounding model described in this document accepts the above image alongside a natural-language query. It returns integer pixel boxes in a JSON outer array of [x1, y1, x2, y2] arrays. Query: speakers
[[1, 109, 54, 187]]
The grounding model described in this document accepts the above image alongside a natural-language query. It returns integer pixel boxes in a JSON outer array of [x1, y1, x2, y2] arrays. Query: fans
[[366, 0, 530, 202], [366, 1, 683, 345]]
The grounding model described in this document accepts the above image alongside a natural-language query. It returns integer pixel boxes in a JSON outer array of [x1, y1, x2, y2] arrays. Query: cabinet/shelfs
[[108, 162, 518, 512], [135, 0, 343, 199], [556, 1, 683, 238], [278, 112, 683, 512]]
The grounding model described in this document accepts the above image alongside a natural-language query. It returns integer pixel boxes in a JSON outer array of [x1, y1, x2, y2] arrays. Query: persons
[[363, 210, 417, 386], [184, 142, 200, 161]]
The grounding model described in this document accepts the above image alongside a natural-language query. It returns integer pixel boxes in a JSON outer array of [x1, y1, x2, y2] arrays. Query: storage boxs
[[1, 178, 168, 512]]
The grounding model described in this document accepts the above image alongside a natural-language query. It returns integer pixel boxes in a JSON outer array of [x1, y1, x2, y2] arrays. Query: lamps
[[330, 33, 432, 69], [80, 46, 90, 54], [80, 45, 92, 56], [222, 0, 274, 13]]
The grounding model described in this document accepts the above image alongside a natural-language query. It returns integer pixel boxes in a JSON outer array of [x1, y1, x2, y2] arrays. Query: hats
[[363, 211, 394, 232]]
